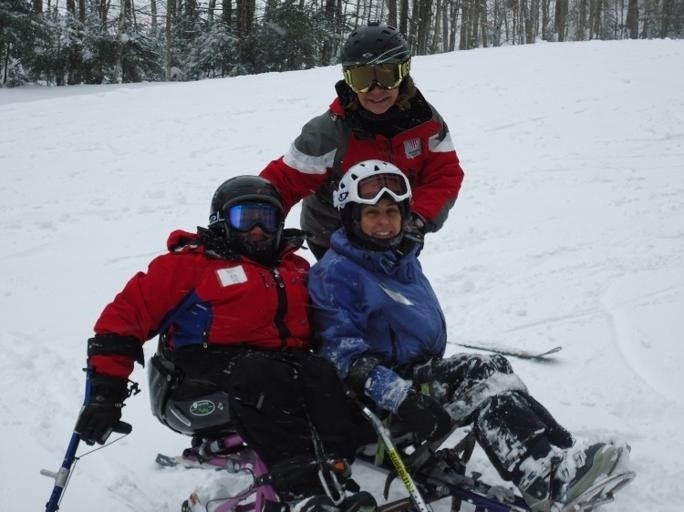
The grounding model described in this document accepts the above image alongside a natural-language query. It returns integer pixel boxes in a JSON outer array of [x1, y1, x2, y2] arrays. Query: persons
[[305, 158, 639, 509], [168, 20, 464, 276], [73, 173, 400, 509]]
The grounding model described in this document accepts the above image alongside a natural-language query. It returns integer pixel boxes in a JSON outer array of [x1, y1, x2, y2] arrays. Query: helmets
[[333, 158, 412, 219], [209, 174, 287, 230], [340, 22, 412, 74]]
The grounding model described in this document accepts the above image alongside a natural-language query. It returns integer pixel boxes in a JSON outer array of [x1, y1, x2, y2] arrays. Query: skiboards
[[447, 340, 561, 359]]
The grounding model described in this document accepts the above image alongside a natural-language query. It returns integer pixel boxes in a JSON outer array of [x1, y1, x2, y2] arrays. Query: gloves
[[395, 213, 426, 260], [397, 380, 451, 442], [75, 372, 129, 446]]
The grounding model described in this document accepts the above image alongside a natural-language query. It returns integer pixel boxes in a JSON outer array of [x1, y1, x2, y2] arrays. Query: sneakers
[[268, 483, 344, 512], [314, 479, 377, 512], [552, 431, 632, 500], [519, 440, 615, 509]]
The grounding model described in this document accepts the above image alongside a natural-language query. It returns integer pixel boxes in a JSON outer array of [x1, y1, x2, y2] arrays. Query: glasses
[[226, 203, 283, 236], [342, 62, 404, 95], [351, 170, 412, 202]]
